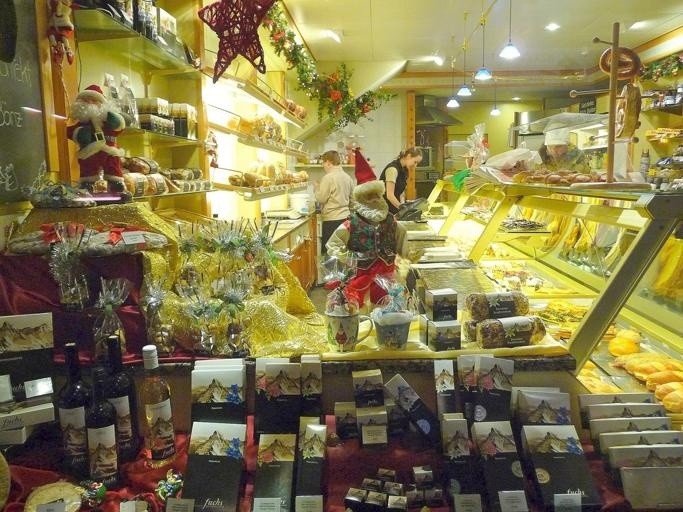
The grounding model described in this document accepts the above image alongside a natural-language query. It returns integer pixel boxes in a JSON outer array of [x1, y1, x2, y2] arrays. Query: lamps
[[475, 23, 493, 81], [447, 63, 462, 109], [499, 1, 521, 60], [457, 47, 475, 96]]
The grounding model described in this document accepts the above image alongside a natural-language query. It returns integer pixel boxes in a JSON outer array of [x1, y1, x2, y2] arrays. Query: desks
[[48, 322, 577, 437]]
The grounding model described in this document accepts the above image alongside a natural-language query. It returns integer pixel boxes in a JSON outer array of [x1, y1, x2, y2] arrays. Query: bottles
[[586, 151, 607, 169], [654, 178, 661, 188], [642, 83, 683, 112], [58, 341, 93, 474], [639, 148, 650, 178], [104, 334, 139, 461], [86, 365, 121, 488], [135, 344, 177, 469], [661, 177, 670, 191]]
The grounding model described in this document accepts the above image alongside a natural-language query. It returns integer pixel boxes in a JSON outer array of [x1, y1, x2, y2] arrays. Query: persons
[[313, 149, 355, 263], [345, 143, 377, 186], [66, 84, 127, 194], [324, 147, 408, 314], [379, 147, 424, 214], [47, 0, 75, 66]]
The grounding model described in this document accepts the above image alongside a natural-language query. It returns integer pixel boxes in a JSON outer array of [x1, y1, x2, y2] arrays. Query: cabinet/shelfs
[[67, 0, 219, 224], [629, 52, 683, 192], [422, 177, 683, 432], [203, 1, 318, 294]]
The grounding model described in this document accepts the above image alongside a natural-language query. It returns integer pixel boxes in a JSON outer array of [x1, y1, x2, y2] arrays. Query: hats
[[77, 85, 107, 103], [352, 148, 385, 199]]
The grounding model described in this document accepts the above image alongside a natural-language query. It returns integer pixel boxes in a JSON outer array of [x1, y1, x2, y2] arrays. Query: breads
[[257, 175, 271, 186], [167, 179, 184, 193], [555, 169, 570, 177], [592, 170, 607, 181], [160, 168, 171, 178], [568, 172, 590, 182], [205, 179, 213, 191], [514, 170, 530, 182], [121, 167, 129, 173], [647, 371, 682, 391], [128, 156, 159, 174], [663, 390, 683, 413], [183, 169, 193, 180], [296, 103, 307, 117], [530, 169, 550, 183], [147, 173, 169, 194], [617, 328, 639, 343], [229, 114, 283, 141], [612, 352, 671, 360], [196, 180, 205, 190], [285, 99, 296, 111], [634, 359, 683, 380], [122, 156, 129, 166], [655, 381, 683, 400], [229, 174, 245, 185], [246, 164, 309, 186], [608, 338, 639, 355], [193, 168, 202, 178], [626, 358, 664, 374], [184, 182, 191, 192], [466, 291, 529, 320], [545, 173, 561, 183], [125, 173, 148, 197], [190, 180, 197, 191], [172, 169, 183, 180], [463, 319, 479, 343], [478, 315, 547, 349]]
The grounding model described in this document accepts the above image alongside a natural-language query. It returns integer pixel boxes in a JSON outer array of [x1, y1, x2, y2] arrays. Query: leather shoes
[[79, 182, 94, 192], [107, 179, 124, 192]]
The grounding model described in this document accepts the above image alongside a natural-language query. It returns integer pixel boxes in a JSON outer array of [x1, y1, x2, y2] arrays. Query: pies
[[536, 300, 587, 337], [603, 322, 618, 337]]
